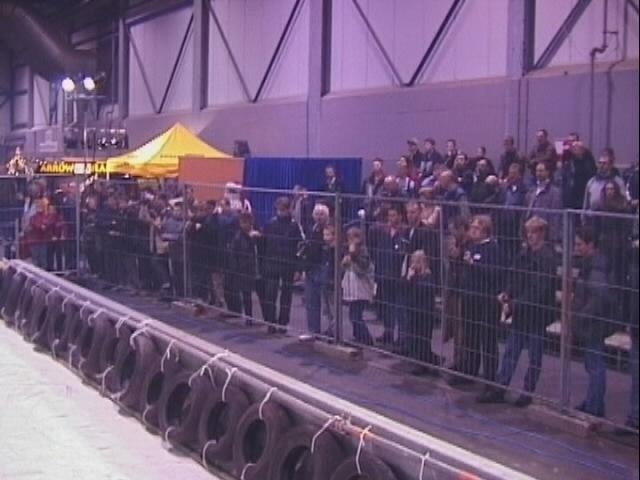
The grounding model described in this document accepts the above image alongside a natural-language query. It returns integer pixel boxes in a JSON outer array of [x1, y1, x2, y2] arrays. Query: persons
[[288, 128, 637, 440], [16, 174, 314, 337]]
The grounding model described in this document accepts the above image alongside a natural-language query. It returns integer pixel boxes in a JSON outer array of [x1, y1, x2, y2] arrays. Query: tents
[[102, 122, 245, 201]]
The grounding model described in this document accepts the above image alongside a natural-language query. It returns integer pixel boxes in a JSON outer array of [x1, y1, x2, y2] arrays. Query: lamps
[[61, 72, 85, 93], [83, 71, 108, 91]]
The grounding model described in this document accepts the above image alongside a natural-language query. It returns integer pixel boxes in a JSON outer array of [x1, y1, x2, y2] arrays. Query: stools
[[604, 331, 632, 353], [546, 319, 561, 338]]
